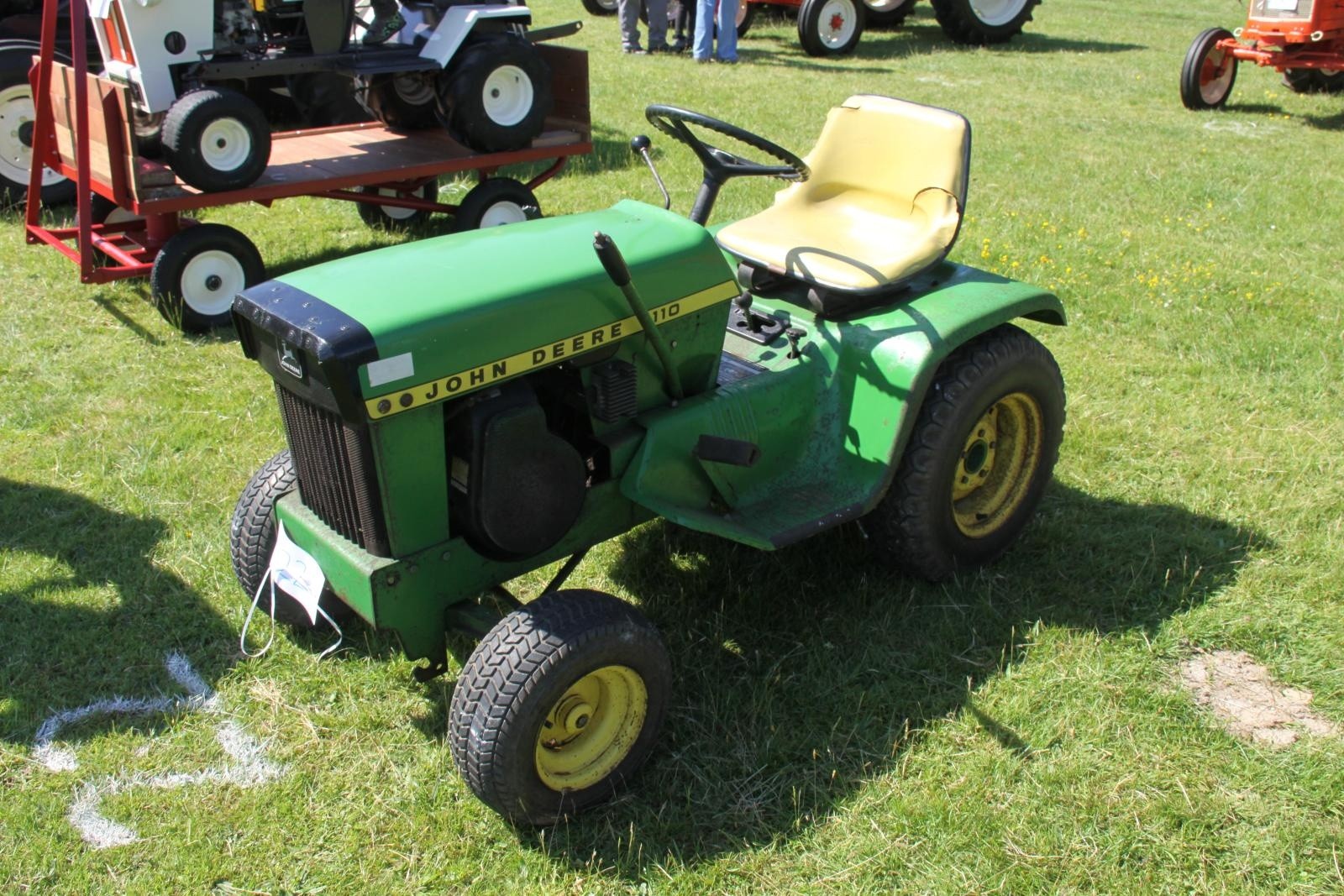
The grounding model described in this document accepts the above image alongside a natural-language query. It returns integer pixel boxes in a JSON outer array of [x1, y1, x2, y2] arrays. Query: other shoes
[[649, 43, 680, 53], [626, 46, 647, 56], [674, 37, 687, 47]]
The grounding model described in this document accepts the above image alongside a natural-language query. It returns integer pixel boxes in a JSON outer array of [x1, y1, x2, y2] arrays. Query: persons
[[672, 0, 696, 49], [362, 0, 406, 45], [693, 0, 739, 62], [618, 0, 682, 54]]
